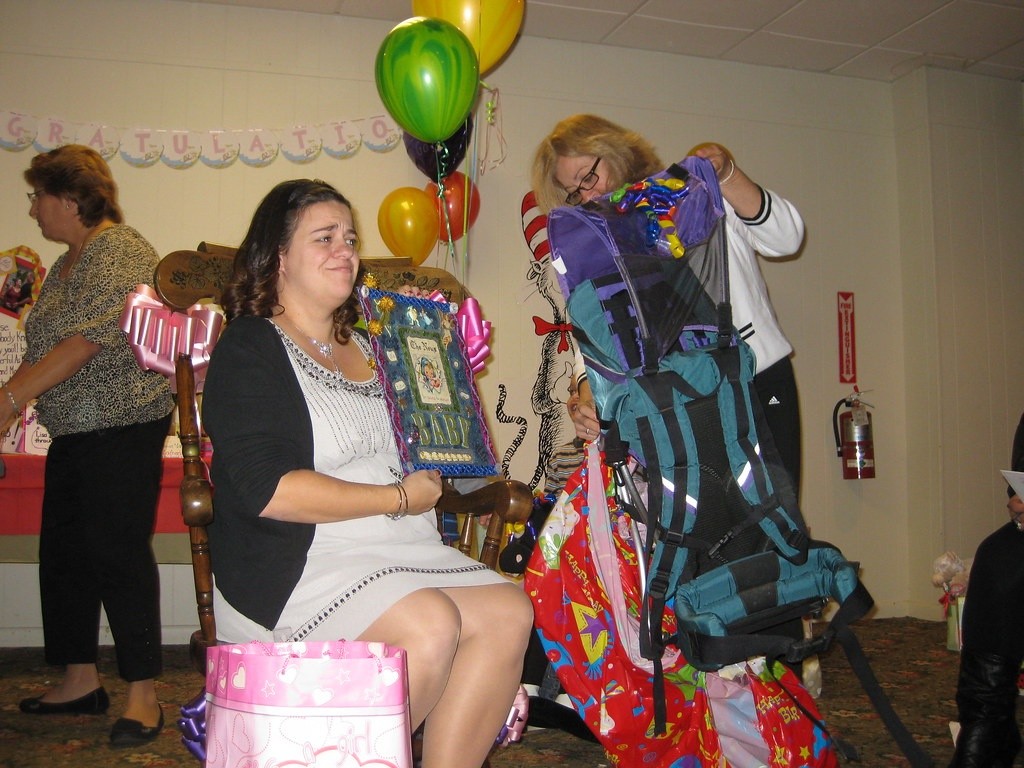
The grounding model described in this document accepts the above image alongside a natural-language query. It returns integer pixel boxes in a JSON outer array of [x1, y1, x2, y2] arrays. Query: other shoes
[[19, 686, 109, 713], [109, 703, 163, 748]]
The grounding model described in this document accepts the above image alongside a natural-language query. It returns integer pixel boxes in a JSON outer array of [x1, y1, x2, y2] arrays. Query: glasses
[[566, 157, 601, 206], [27, 189, 44, 203]]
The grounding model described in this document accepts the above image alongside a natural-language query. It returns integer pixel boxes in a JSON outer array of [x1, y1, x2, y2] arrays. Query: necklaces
[[277, 309, 348, 380]]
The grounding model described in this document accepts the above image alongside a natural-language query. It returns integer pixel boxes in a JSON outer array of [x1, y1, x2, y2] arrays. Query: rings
[[587, 429, 589, 433], [1018, 523, 1021, 529]]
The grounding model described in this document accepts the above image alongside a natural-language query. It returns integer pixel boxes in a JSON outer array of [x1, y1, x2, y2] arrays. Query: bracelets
[[385, 483, 409, 521], [720, 160, 734, 184], [5, 383, 17, 409]]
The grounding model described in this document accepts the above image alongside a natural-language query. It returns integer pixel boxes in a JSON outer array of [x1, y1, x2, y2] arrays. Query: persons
[[947, 412, 1024, 768], [201, 178, 535, 768], [0, 144, 177, 748], [528, 113, 805, 678]]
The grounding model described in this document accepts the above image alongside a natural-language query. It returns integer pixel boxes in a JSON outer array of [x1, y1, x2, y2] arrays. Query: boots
[[950, 647, 1024, 768]]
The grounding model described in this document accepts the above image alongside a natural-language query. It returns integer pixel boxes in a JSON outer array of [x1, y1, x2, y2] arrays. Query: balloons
[[424, 172, 480, 242], [411, 0, 525, 75], [374, 15, 481, 144], [404, 112, 473, 184], [377, 187, 439, 267]]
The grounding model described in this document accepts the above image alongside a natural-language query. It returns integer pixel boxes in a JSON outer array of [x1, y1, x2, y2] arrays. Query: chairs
[[171, 354, 532, 674]]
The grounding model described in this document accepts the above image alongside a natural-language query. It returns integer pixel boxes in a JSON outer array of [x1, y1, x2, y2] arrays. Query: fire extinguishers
[[832, 385, 875, 481]]
